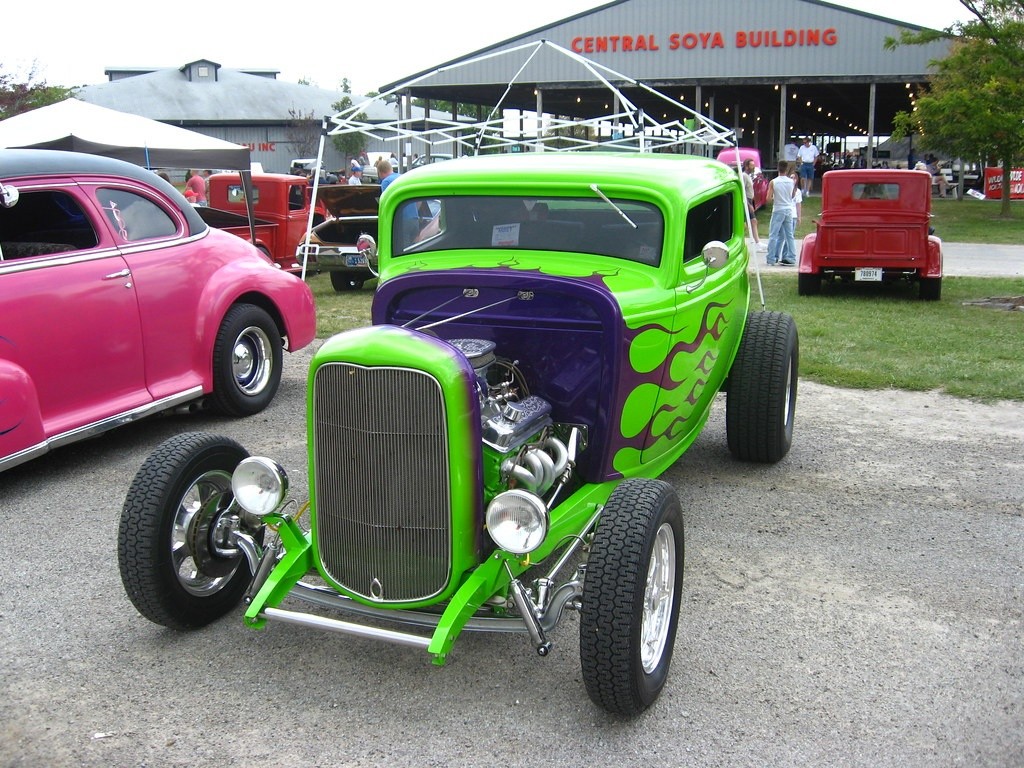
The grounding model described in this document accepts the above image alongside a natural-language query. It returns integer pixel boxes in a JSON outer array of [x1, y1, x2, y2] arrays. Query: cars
[[0, 146, 320, 476], [715, 146, 768, 213], [114, 146, 801, 717], [795, 168, 945, 302], [190, 153, 465, 293]]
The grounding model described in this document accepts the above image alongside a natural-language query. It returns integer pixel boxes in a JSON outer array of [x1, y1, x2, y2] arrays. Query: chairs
[[451, 194, 543, 251]]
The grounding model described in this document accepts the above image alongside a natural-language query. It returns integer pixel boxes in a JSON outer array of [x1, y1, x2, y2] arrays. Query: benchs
[[1, 242, 77, 261], [915, 169, 959, 199]]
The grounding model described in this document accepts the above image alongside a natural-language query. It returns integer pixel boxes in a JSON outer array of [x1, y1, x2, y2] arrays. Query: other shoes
[[779, 259, 795, 265], [756, 242, 769, 250], [806, 192, 810, 197], [930, 214, 935, 218], [950, 185, 956, 191]]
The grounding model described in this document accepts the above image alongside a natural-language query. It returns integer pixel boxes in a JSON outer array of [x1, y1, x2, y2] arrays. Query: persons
[[737, 137, 956, 265], [157, 153, 418, 207]]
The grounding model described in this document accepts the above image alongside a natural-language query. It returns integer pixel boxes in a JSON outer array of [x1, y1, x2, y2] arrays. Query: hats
[[351, 167, 362, 172], [185, 190, 198, 197]]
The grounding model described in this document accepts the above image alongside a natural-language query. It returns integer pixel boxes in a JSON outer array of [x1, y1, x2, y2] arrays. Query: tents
[[0, 98, 258, 246]]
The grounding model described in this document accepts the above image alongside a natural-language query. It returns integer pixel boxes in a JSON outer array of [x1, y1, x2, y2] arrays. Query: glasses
[[803, 141, 808, 144]]
